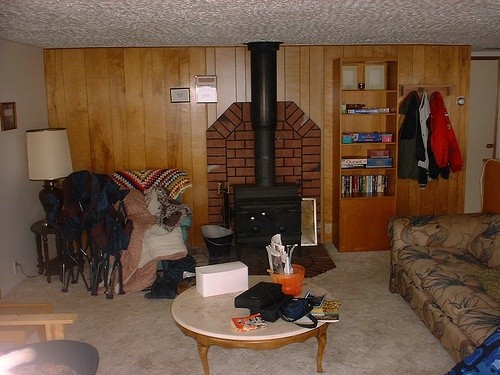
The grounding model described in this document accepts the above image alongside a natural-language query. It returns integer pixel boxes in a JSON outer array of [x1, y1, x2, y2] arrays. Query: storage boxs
[[196, 262, 248, 296]]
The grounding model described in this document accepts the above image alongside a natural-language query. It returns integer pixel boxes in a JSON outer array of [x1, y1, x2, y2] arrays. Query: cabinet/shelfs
[[333, 56, 397, 252]]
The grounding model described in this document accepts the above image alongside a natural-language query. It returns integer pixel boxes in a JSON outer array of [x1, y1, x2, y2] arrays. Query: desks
[[171, 275, 333, 375], [32, 218, 63, 283]]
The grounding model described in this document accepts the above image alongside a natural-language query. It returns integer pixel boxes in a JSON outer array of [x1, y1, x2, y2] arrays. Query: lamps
[[27, 127, 73, 228]]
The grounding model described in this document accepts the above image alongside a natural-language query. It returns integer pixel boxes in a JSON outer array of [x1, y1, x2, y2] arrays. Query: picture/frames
[[0, 102, 17, 131], [170, 88, 190, 102], [195, 75, 218, 103]]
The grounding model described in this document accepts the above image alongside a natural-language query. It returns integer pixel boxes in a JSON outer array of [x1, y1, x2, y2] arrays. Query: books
[[265, 233, 294, 275], [341, 174, 389, 193]]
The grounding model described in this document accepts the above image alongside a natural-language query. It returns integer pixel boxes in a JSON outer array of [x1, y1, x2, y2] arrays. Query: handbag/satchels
[[235, 282, 283, 308], [280, 298, 318, 328], [251, 294, 294, 321]]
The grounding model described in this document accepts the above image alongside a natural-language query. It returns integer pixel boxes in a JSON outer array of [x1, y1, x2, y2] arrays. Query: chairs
[[0, 313, 99, 375]]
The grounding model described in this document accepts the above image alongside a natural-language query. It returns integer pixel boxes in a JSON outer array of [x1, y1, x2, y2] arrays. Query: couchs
[[390, 213, 500, 363]]
